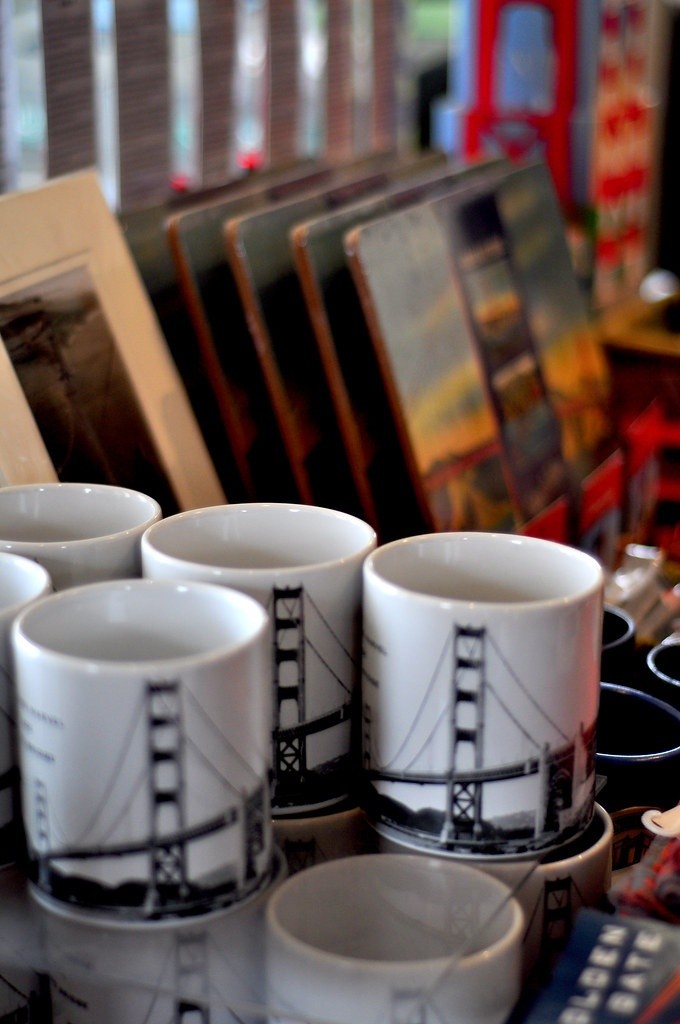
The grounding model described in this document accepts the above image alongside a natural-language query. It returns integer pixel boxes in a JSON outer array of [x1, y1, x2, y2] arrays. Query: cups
[[645, 637, 680, 711], [10, 579, 273, 922], [362, 532, 604, 859], [0, 552, 53, 829], [0, 803, 616, 1024], [0, 482, 163, 593], [601, 609, 637, 682], [140, 502, 376, 816], [595, 681, 680, 813]]
